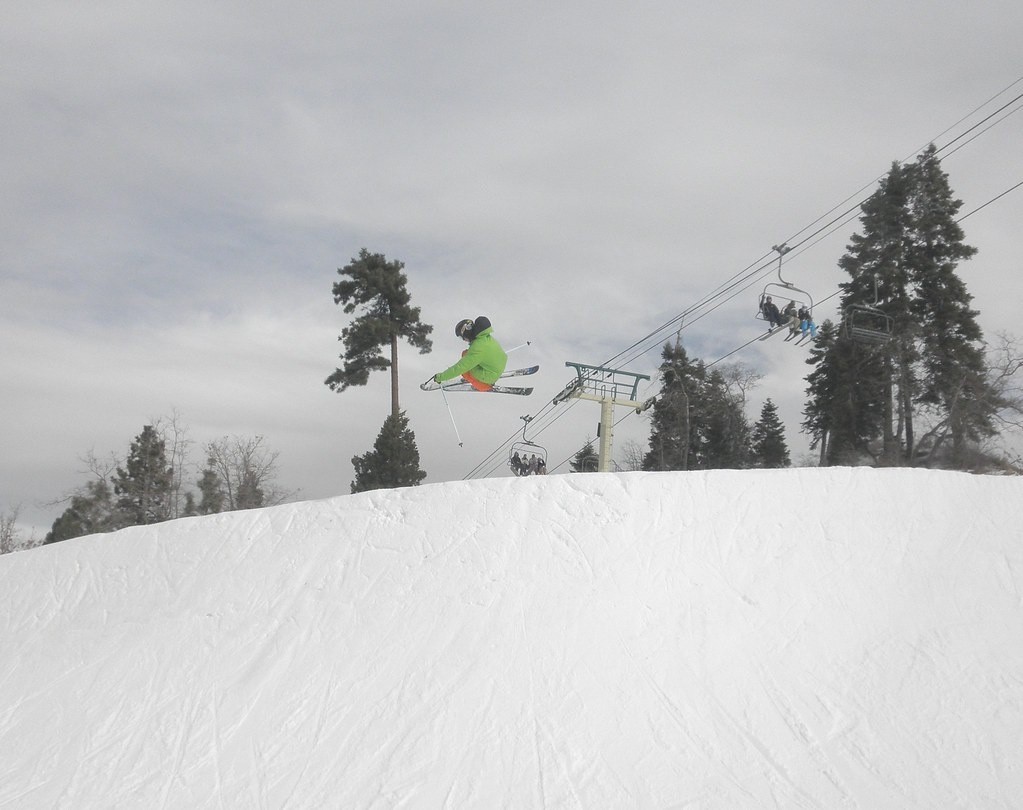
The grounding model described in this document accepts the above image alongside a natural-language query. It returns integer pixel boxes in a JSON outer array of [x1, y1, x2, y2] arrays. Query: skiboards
[[421, 365, 539, 395], [760, 317, 823, 347]]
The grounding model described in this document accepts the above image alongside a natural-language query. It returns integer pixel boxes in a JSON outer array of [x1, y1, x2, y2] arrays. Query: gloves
[[434, 373, 441, 383]]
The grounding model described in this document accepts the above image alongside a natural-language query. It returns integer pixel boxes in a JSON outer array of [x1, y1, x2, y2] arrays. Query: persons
[[434, 316, 508, 392], [760, 295, 782, 328], [511, 452, 547, 476], [798, 305, 817, 337], [785, 300, 802, 332]]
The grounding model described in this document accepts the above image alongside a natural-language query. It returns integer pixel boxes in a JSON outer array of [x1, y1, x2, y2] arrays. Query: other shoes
[[471, 385, 477, 390]]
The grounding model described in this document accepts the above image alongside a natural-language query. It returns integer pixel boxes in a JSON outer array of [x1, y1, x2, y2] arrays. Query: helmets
[[455, 319, 475, 340]]
